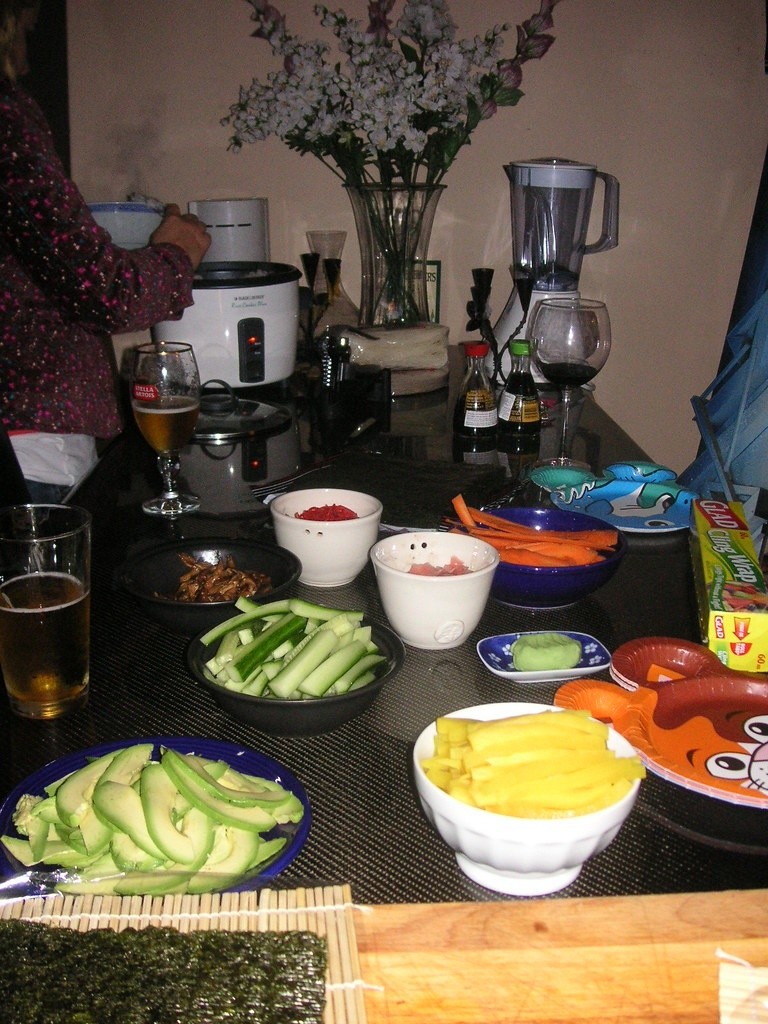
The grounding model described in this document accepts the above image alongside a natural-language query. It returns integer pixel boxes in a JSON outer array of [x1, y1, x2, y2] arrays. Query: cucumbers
[[200, 594, 387, 699]]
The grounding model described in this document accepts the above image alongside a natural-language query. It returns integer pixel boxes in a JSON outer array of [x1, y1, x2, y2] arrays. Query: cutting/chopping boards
[[320, 882, 768, 1024]]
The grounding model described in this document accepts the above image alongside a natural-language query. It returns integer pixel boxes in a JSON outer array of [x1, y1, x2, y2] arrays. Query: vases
[[341, 182, 448, 331]]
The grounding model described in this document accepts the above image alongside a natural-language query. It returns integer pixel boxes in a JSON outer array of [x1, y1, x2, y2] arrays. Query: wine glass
[[128, 343, 204, 516], [529, 299, 612, 473]]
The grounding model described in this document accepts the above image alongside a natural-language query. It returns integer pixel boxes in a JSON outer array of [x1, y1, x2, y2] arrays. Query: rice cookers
[[140, 254, 301, 393], [170, 385, 305, 516]]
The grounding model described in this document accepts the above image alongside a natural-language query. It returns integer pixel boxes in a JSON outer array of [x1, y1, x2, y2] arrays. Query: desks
[[0, 345, 768, 905]]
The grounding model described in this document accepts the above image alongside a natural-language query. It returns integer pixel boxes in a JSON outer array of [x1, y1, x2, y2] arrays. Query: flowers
[[222, 0, 556, 323]]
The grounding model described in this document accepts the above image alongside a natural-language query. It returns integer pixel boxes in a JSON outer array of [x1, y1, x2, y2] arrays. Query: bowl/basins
[[270, 487, 384, 588], [369, 531, 501, 651], [412, 701, 645, 896], [179, 609, 408, 734], [126, 534, 302, 640], [79, 201, 174, 250], [451, 505, 626, 610]]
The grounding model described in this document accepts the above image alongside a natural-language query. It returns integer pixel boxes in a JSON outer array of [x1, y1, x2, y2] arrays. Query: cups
[[0, 502, 91, 720]]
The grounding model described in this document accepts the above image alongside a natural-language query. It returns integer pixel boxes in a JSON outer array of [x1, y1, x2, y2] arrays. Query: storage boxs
[[688, 500, 768, 672]]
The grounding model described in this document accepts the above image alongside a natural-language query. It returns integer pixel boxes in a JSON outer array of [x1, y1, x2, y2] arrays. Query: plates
[[476, 629, 611, 683], [0, 736, 310, 904], [548, 638, 767, 819], [533, 460, 702, 532]]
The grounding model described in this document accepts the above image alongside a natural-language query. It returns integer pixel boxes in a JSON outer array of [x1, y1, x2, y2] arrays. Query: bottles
[[307, 230, 360, 338], [497, 339, 544, 433], [451, 341, 497, 437]]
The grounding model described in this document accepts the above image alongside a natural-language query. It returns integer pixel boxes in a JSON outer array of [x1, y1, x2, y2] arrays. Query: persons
[[0, 0, 210, 555]]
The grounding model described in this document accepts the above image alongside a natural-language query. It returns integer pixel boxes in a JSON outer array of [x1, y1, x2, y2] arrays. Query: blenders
[[474, 154, 619, 392]]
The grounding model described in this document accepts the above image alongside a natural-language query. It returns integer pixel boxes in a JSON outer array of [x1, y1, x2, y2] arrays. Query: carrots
[[444, 494, 618, 567]]
